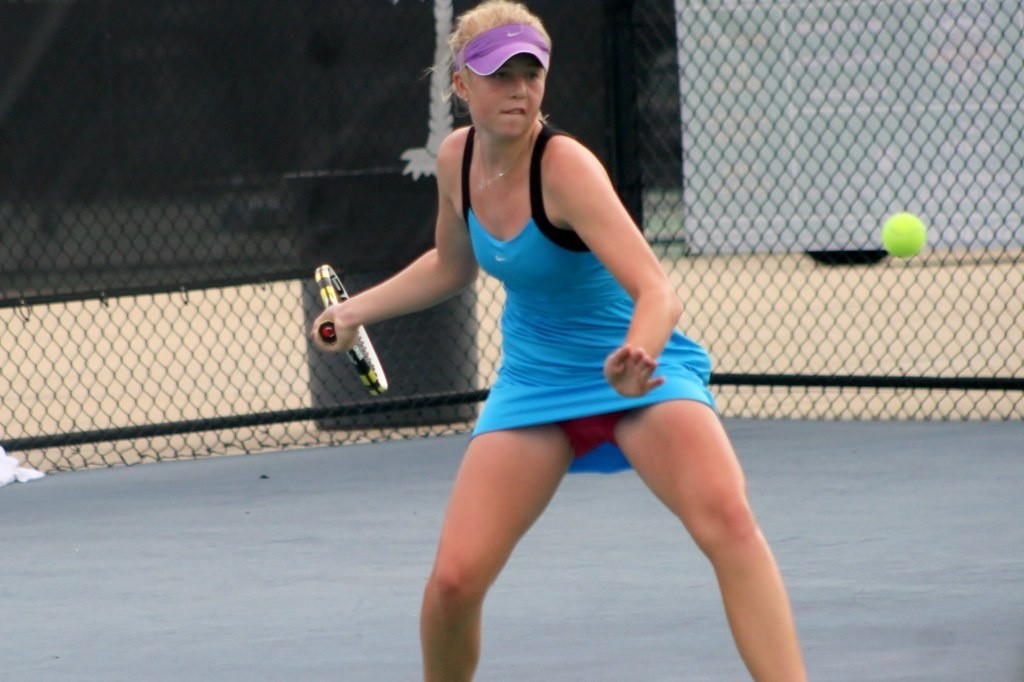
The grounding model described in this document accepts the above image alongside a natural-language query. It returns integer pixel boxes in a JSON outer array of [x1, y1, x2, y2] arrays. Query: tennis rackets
[[312, 263, 389, 397]]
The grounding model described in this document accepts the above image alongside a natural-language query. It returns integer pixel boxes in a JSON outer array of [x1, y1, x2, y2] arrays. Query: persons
[[307, 1, 818, 681]]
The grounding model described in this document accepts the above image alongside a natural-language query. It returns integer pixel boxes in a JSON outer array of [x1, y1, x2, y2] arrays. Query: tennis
[[880, 212, 928, 258]]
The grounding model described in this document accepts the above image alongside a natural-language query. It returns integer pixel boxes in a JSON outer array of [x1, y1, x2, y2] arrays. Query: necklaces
[[476, 124, 539, 194]]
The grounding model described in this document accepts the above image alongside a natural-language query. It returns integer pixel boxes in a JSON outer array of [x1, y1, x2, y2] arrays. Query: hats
[[454, 24, 552, 75]]
